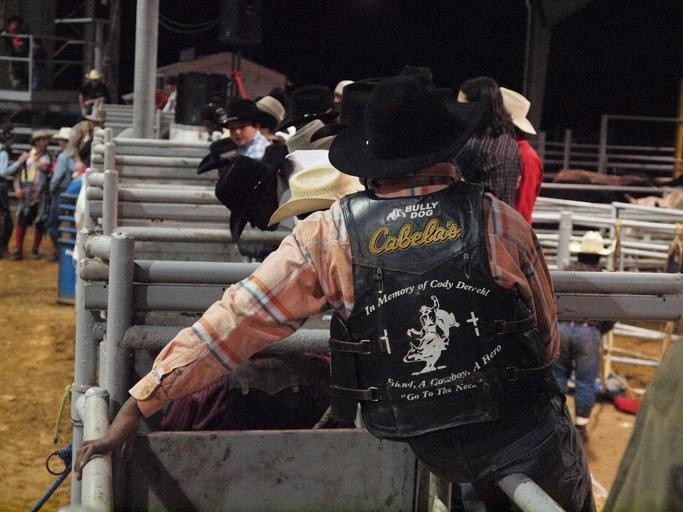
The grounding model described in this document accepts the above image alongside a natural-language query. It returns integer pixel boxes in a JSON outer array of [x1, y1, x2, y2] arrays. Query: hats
[[1, 108, 106, 143], [568, 231, 617, 256], [84, 69, 104, 80]]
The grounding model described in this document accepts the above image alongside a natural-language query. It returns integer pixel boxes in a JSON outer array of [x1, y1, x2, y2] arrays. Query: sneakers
[[576, 425, 588, 443], [12, 250, 22, 260], [32, 249, 40, 259]]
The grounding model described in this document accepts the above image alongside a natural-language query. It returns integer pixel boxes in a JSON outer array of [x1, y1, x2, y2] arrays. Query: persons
[[78, 68, 112, 123], [155, 77, 177, 140], [451, 76, 522, 208], [74, 119, 104, 174], [499, 86, 545, 227], [554, 231, 615, 443], [201, 66, 436, 244], [1, 125, 89, 263], [73, 76, 597, 512], [1, 14, 59, 90]]
